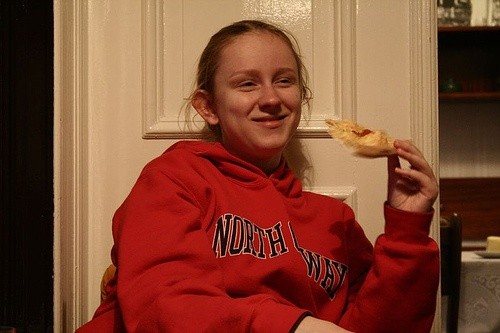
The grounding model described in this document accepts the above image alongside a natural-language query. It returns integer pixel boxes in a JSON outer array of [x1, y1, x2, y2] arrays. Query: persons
[[73, 19, 439, 333]]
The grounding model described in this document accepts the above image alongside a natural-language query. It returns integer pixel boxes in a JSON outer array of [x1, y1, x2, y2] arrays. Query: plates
[[474, 251, 500, 258]]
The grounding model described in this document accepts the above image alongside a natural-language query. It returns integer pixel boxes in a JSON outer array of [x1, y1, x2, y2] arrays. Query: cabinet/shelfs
[[438, 26, 500, 100]]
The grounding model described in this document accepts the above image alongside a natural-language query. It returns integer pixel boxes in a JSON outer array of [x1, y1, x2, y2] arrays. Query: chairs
[[440, 213, 462, 333]]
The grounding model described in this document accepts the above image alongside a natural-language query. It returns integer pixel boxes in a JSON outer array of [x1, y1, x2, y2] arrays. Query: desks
[[442, 251, 500, 333]]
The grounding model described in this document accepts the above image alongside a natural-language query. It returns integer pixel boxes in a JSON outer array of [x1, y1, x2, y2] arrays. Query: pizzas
[[325, 119, 414, 156]]
